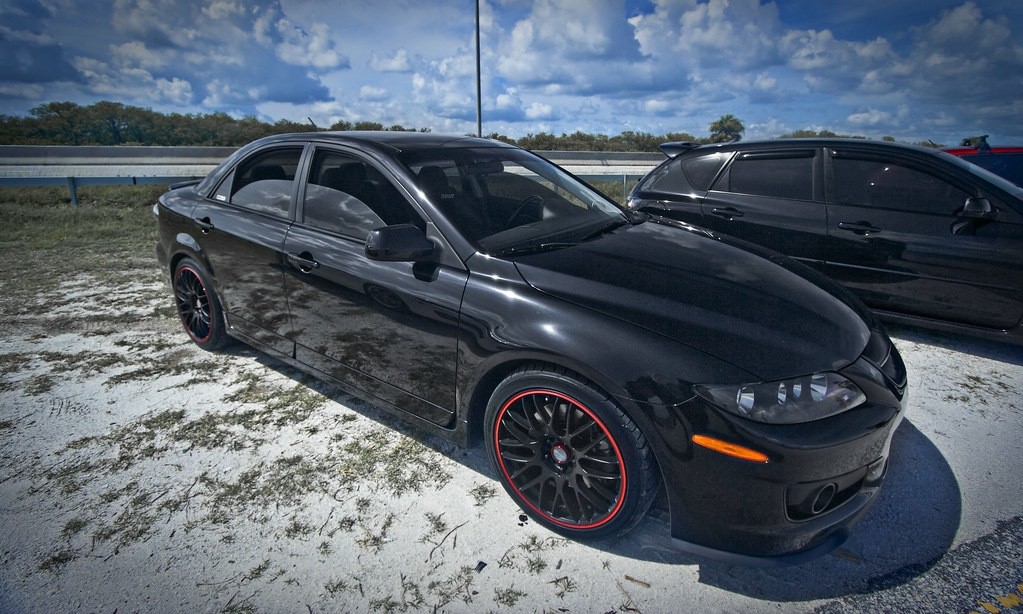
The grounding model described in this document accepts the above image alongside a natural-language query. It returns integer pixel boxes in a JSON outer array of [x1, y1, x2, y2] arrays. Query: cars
[[153, 129, 913, 558], [863, 142, 1023, 207], [619, 133, 1023, 349]]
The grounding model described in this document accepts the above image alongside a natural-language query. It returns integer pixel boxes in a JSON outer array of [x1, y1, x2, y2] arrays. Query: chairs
[[417, 165, 475, 240], [249, 165, 287, 184], [334, 161, 390, 226], [320, 167, 340, 189]]
